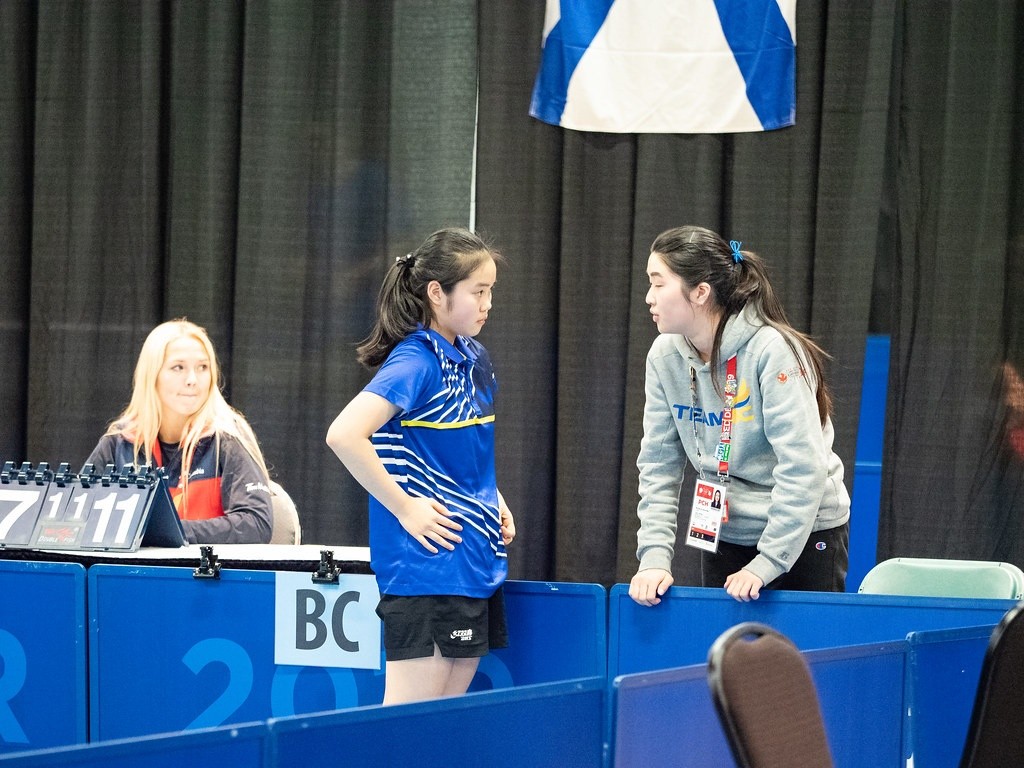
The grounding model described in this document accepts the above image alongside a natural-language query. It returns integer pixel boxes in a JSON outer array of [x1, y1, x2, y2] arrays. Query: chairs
[[856, 555, 1024, 601], [960, 599, 1024, 768], [268, 481, 302, 545], [705, 621, 838, 768]]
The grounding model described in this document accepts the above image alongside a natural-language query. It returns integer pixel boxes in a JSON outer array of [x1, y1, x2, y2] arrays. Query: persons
[[327, 226, 519, 708], [78, 317, 275, 547], [629, 223, 851, 606]]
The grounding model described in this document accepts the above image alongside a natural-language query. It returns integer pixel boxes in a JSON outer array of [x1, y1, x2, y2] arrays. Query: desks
[[28, 543, 374, 563]]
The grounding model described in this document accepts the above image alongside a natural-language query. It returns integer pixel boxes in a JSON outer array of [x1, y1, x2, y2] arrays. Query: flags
[[527, 0, 796, 135]]
